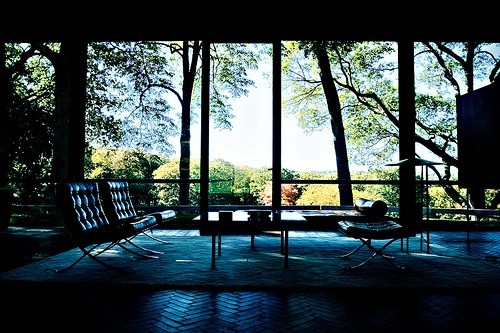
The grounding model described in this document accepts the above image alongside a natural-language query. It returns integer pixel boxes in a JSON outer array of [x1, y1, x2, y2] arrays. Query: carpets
[[0, 234, 500, 302]]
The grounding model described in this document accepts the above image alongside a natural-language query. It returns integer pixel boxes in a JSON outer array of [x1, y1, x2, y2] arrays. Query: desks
[[192, 209, 308, 267]]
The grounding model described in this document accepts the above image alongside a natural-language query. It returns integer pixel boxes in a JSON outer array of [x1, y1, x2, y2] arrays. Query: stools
[[336, 220, 411, 273]]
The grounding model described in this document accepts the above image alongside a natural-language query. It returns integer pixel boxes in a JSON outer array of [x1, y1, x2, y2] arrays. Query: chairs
[[54, 180, 177, 275]]
